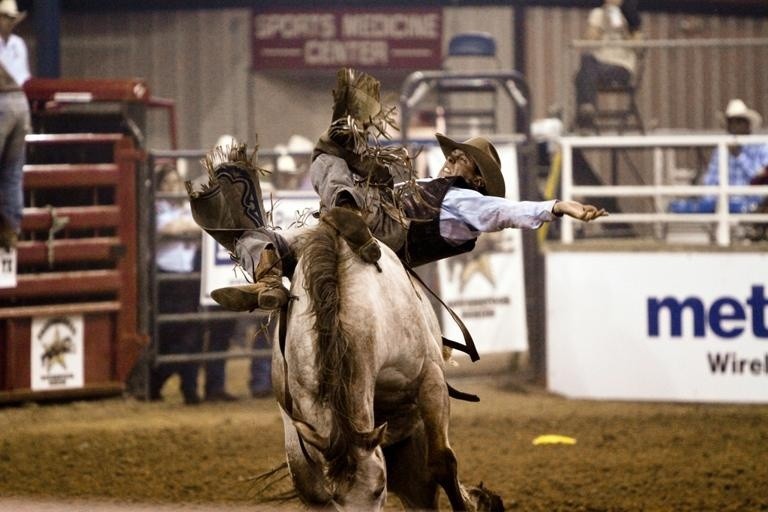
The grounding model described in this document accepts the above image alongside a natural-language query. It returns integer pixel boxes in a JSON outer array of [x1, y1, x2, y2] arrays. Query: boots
[[209, 249, 290, 313]]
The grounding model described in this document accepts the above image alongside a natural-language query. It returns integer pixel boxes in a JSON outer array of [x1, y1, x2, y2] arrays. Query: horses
[[225, 219, 505, 512]]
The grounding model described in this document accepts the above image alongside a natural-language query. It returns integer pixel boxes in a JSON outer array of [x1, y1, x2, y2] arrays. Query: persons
[[574, 0, 647, 130], [207, 133, 612, 312], [150, 132, 316, 406], [1, 1, 32, 89], [1, 63, 32, 252], [697, 97, 768, 242]]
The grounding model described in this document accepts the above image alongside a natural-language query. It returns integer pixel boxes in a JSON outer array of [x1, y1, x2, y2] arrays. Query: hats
[[434, 132, 506, 199], [0, 0, 27, 23], [713, 99, 763, 134]]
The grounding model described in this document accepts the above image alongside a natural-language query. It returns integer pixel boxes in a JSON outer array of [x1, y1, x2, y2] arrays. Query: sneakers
[[142, 380, 276, 404], [330, 207, 381, 264]]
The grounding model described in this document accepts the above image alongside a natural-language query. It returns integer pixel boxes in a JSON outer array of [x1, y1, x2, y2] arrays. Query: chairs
[[432, 33, 501, 132], [574, 31, 647, 135]]
